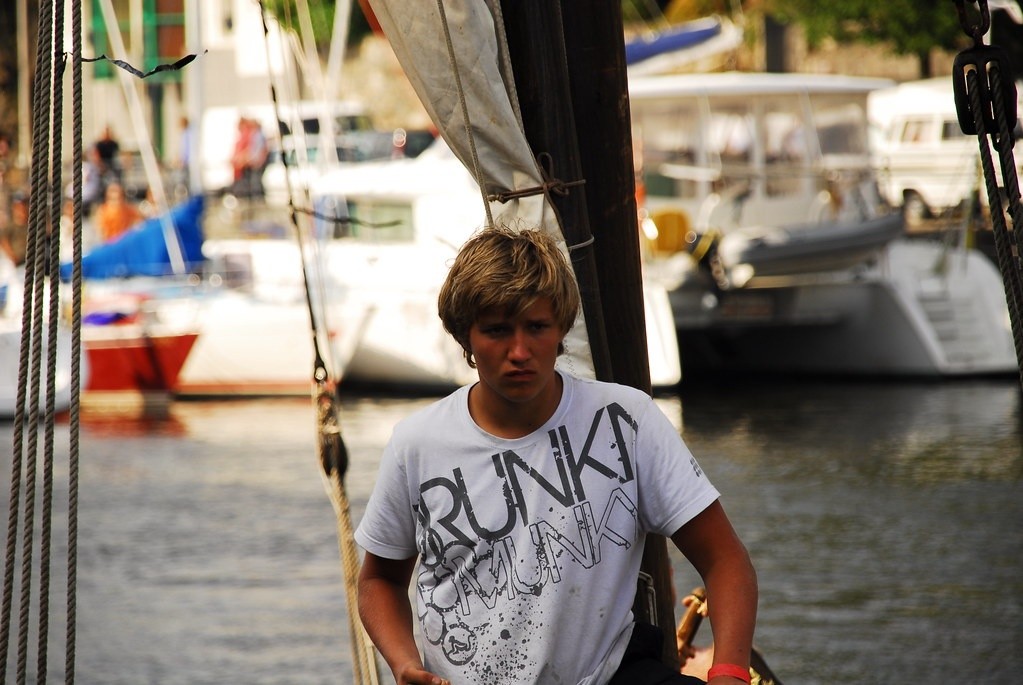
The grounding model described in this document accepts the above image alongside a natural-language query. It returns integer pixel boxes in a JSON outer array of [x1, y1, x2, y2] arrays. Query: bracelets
[[707, 664, 751, 684]]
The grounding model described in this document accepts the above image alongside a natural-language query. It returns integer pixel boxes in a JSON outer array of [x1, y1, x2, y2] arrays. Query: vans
[[877, 78, 1023, 229]]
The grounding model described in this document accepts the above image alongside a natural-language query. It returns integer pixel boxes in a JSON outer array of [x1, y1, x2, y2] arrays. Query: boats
[[0, 0, 1019, 419]]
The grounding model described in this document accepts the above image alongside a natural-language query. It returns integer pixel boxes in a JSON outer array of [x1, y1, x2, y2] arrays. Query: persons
[[82, 127, 143, 241], [232, 116, 267, 196], [353, 230, 759, 685]]
